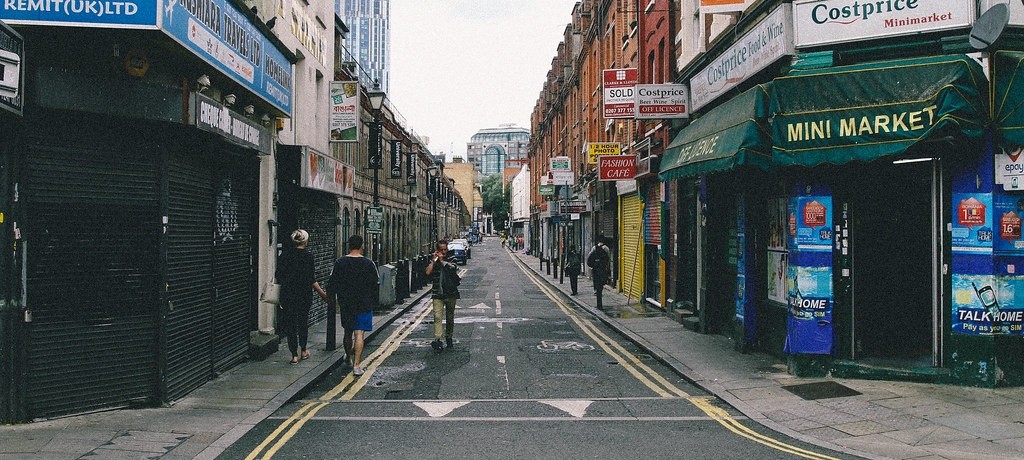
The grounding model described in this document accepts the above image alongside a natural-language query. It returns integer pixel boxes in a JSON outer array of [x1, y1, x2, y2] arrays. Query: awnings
[[659, 51, 1024, 183]]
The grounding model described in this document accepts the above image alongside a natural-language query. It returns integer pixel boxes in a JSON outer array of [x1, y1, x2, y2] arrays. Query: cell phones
[[972, 282, 1001, 325]]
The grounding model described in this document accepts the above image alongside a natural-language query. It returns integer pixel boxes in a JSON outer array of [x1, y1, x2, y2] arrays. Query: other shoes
[[447, 339, 453, 348], [431, 340, 444, 351], [343, 354, 353, 367], [354, 367, 365, 376]]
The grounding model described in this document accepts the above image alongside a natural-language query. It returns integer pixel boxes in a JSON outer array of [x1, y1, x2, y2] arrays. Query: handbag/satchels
[[260, 275, 282, 305]]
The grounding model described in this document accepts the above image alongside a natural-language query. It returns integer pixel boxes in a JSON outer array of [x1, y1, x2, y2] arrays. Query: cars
[[446, 237, 472, 265]]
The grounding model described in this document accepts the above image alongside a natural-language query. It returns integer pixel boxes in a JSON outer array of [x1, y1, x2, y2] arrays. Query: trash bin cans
[[378, 263, 396, 305], [395, 274, 404, 305]]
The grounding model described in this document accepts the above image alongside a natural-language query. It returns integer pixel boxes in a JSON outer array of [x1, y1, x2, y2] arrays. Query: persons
[[566, 245, 581, 296], [274, 229, 381, 375], [588, 234, 610, 296], [425, 240, 461, 349], [478, 232, 482, 241], [508, 233, 524, 251]]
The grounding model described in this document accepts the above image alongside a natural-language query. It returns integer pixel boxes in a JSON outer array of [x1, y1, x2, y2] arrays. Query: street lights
[[426, 165, 439, 255], [362, 76, 388, 312]]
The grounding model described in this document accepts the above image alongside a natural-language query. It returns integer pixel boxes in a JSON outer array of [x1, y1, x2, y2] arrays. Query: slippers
[[301, 350, 310, 359], [289, 359, 298, 365]]
